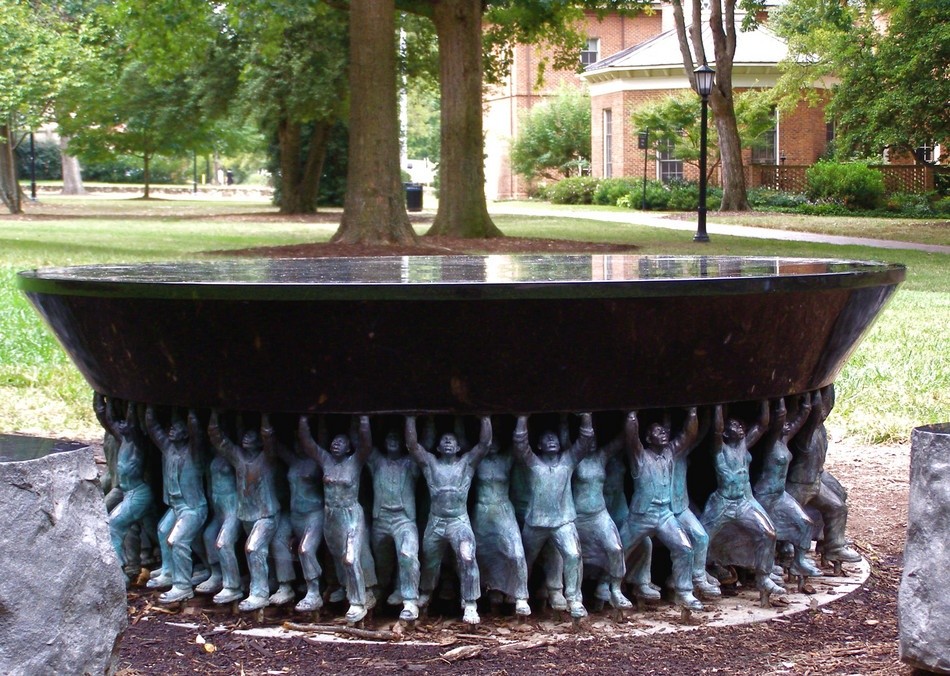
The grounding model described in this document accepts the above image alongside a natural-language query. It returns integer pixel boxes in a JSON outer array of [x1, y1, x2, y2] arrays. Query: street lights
[[690, 63, 717, 243], [24, 123, 39, 199]]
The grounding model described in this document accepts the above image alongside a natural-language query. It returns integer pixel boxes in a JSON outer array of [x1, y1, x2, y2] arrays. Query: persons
[[90, 383, 861, 625], [218, 168, 233, 186]]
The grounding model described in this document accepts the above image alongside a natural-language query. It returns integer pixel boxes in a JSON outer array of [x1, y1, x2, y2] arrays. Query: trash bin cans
[[406, 187, 423, 212]]
